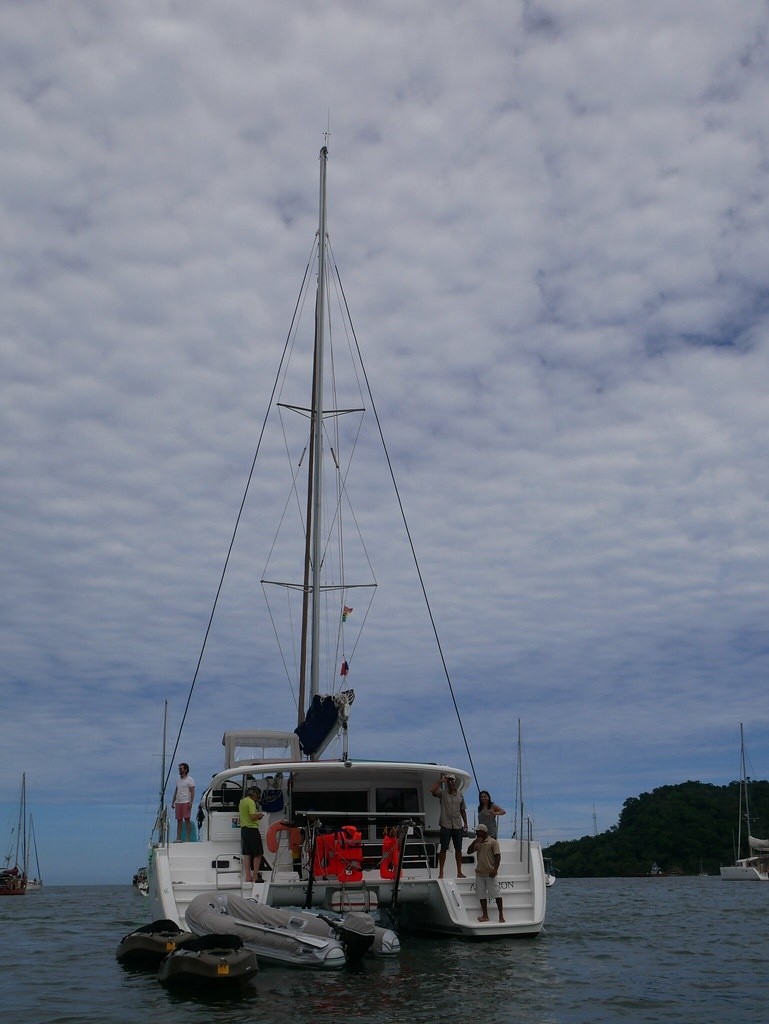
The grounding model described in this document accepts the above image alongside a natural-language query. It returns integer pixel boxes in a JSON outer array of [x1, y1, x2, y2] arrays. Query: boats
[[116, 918, 261, 996], [186, 887, 401, 970]]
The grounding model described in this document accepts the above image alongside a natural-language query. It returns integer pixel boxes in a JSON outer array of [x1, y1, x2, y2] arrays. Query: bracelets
[[497, 811, 498, 814], [190, 802, 193, 804]]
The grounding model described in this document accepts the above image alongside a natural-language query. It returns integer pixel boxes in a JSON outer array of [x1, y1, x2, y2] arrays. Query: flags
[[340, 661, 349, 676], [342, 606, 353, 622]]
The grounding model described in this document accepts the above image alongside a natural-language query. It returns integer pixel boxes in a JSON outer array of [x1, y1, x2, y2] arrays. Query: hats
[[473, 824, 487, 832], [248, 786, 261, 799], [445, 774, 455, 779]]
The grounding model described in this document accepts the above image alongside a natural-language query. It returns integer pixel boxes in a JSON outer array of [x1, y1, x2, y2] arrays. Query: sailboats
[[131, 144, 547, 940], [718, 721, 769, 881], [0, 771, 43, 895]]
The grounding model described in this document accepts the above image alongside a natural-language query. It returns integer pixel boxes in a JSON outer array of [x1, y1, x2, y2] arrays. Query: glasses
[[179, 768, 182, 770], [448, 780, 455, 784]]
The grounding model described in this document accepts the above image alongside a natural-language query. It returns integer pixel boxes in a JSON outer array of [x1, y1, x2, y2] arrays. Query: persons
[[171, 763, 195, 843], [478, 791, 506, 840], [467, 824, 505, 923], [239, 786, 266, 882], [431, 774, 468, 879]]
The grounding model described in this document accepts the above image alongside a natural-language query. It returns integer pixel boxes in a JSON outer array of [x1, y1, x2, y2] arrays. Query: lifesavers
[[265, 818, 301, 854]]
